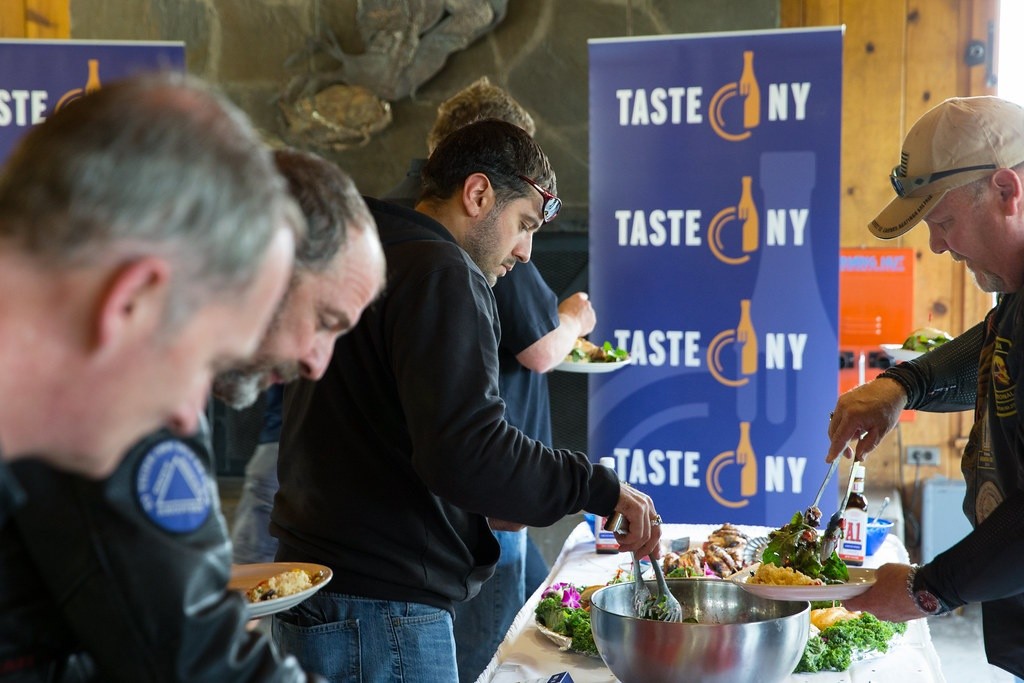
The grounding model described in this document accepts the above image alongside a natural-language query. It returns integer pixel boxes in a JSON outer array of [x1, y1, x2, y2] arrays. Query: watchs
[[913, 566, 949, 617]]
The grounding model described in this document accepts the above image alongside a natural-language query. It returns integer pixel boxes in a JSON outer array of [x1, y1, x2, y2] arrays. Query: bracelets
[[906, 563, 919, 600]]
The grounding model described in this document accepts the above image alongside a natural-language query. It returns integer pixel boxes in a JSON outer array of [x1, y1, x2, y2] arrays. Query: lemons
[[579, 586, 606, 599]]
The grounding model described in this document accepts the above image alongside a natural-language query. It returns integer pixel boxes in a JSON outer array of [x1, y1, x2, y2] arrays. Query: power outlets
[[907, 447, 939, 464]]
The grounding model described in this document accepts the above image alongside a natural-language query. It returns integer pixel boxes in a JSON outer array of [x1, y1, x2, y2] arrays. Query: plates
[[557, 354, 631, 374], [880, 340, 928, 362], [223, 563, 334, 620], [733, 564, 879, 602]]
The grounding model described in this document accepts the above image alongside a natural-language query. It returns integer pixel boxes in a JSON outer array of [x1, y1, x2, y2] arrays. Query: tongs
[[614, 512, 683, 624], [775, 433, 870, 560]]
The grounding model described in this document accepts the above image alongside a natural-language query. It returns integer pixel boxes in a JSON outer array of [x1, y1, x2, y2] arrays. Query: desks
[[473, 522, 943, 683]]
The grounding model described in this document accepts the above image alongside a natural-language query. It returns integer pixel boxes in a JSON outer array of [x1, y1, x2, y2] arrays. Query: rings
[[830, 411, 833, 420], [651, 514, 662, 527]]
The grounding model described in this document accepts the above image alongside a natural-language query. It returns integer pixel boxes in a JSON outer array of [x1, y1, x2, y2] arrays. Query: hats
[[866, 96, 1024, 238]]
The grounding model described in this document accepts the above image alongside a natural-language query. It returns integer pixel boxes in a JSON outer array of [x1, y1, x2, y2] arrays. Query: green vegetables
[[535, 583, 599, 655], [761, 510, 850, 584], [641, 597, 698, 622], [666, 566, 704, 577], [795, 599, 907, 672], [570, 341, 628, 363]]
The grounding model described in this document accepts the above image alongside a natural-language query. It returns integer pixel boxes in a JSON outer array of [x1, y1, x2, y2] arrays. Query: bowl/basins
[[590, 574, 812, 683], [866, 518, 894, 558]]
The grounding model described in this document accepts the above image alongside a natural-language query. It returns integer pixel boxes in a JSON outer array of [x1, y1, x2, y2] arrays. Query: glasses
[[889, 164, 996, 199], [491, 165, 563, 226]]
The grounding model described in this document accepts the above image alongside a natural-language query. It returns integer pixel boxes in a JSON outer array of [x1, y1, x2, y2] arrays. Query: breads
[[809, 607, 860, 629], [245, 568, 314, 603]]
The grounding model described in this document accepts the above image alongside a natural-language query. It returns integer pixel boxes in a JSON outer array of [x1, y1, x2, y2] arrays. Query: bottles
[[838, 466, 868, 566]]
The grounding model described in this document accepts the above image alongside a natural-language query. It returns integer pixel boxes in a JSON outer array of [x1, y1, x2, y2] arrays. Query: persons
[[0, 73, 663, 683], [826, 95, 1024, 683]]
